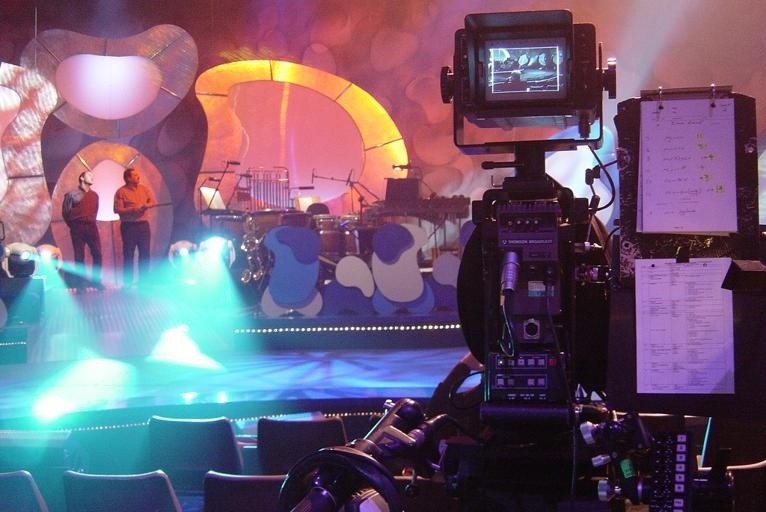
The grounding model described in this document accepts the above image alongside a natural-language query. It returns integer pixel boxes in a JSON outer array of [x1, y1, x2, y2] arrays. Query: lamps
[[169, 238, 236, 276], [1, 241, 64, 281]]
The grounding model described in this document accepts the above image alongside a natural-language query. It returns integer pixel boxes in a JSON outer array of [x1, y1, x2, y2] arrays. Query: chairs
[[61, 470, 184, 512], [202, 468, 290, 512], [148, 414, 245, 495], [256, 416, 349, 476], [0, 470, 49, 511]]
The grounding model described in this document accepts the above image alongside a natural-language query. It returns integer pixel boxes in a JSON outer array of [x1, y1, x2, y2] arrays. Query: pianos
[[361, 195, 469, 225]]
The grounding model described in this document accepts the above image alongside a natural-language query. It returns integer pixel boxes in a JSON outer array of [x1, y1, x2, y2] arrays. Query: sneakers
[[75, 283, 106, 296]]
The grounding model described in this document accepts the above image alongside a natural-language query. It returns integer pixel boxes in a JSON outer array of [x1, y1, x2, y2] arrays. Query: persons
[[500, 70, 529, 91], [62, 170, 107, 293], [426, 351, 483, 419], [113, 168, 156, 290]]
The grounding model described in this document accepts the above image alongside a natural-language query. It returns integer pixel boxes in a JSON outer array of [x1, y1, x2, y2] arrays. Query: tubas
[[240, 216, 263, 284]]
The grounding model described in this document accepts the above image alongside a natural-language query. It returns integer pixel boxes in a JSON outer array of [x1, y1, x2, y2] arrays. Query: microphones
[[237, 173, 253, 178], [223, 159, 241, 165], [311, 168, 315, 184]]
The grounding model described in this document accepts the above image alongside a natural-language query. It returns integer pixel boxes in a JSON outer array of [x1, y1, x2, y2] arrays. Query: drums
[[338, 215, 359, 222], [317, 230, 343, 255], [345, 230, 365, 255], [316, 216, 336, 230]]
